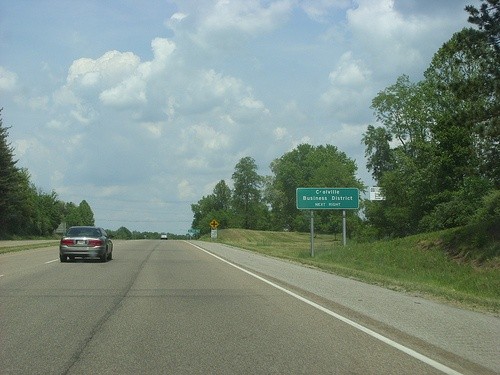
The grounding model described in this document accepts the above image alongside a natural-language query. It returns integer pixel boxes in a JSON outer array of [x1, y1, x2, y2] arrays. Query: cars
[[161, 234, 168, 240], [59, 225, 114, 263]]
